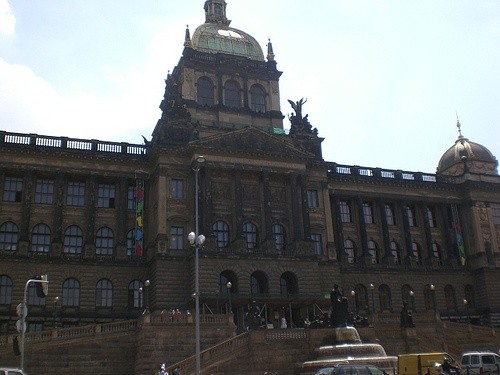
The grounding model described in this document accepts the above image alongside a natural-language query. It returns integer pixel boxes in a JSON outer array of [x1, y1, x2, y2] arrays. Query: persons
[[281, 316, 286, 328], [303, 114, 312, 128], [330, 285, 343, 313], [160, 308, 191, 322], [289, 112, 296, 123], [441, 358, 456, 375], [305, 318, 311, 327]]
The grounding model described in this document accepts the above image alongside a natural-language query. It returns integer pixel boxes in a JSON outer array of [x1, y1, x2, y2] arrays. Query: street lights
[[138, 288, 143, 317], [369, 285, 378, 315], [189, 156, 208, 375], [429, 283, 438, 314], [144, 279, 151, 314], [409, 290, 417, 315], [463, 298, 472, 320], [53, 296, 61, 329], [225, 281, 233, 315], [350, 289, 356, 319]]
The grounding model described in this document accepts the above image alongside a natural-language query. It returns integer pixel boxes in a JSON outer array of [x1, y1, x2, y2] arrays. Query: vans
[[460, 350, 500, 375], [398, 352, 464, 375]]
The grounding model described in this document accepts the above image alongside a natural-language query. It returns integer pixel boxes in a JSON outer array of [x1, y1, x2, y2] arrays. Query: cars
[[314, 362, 390, 375], [0, 366, 27, 375]]
